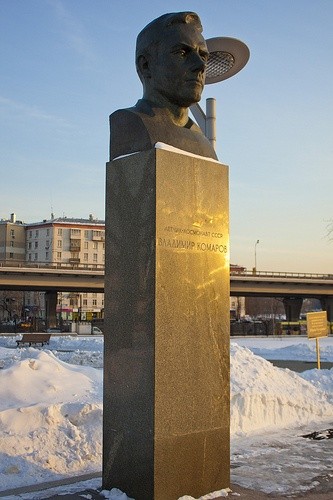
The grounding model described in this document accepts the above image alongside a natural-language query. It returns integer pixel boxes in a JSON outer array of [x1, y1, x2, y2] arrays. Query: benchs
[[16, 334, 51, 347]]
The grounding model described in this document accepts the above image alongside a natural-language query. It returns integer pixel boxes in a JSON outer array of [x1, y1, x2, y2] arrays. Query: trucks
[[69, 323, 103, 335]]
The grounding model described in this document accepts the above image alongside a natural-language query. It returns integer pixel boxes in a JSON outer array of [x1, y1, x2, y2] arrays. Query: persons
[[109, 12, 221, 162]]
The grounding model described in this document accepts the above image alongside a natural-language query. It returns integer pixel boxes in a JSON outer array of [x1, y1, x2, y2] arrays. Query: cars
[[230, 317, 279, 335], [46, 327, 64, 333]]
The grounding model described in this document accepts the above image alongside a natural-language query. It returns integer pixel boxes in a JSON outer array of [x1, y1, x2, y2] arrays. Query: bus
[[275, 320, 333, 336]]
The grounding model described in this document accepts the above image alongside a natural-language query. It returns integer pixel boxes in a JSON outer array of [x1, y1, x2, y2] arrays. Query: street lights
[[11, 298, 25, 324], [255, 240, 260, 273]]
